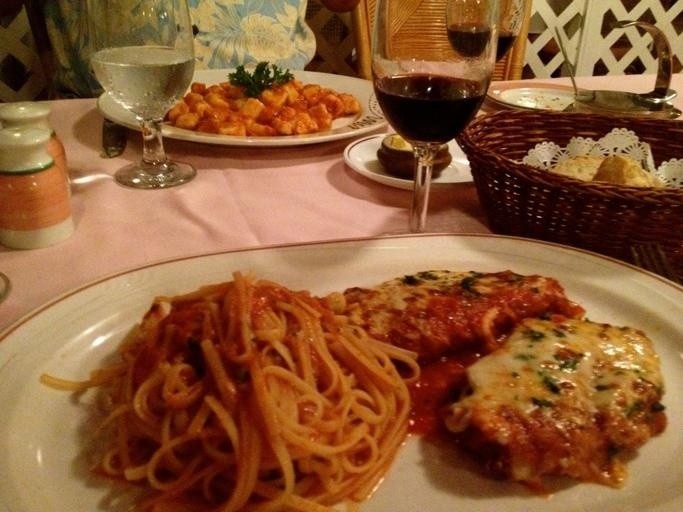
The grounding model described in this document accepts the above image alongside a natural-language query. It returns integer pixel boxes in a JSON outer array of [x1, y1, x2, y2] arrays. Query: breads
[[553, 150, 664, 189]]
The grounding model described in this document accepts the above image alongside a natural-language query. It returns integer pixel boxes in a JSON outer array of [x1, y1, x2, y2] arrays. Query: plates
[[0, 235, 683, 511], [486, 79, 588, 112], [344, 130, 483, 191], [96, 69, 392, 149]]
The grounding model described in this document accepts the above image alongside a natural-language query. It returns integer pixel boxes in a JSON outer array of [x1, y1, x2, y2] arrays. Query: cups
[[0, 101, 76, 247]]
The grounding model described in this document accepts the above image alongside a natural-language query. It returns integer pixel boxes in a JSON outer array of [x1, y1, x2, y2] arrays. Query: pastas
[[39, 271, 419, 512]]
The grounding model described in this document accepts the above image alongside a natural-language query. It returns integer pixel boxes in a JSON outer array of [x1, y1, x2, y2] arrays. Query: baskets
[[455, 108, 683, 271]]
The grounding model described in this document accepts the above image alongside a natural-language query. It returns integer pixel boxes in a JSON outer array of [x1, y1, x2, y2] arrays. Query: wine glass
[[88, 0, 196, 190], [372, 0, 526, 232]]
